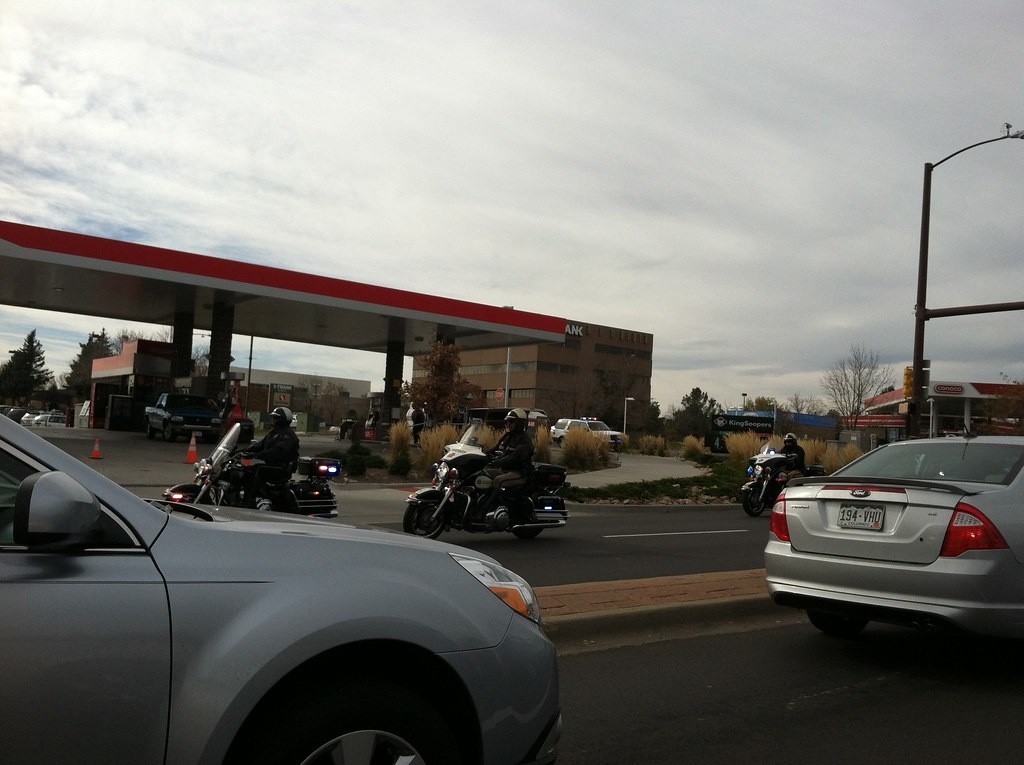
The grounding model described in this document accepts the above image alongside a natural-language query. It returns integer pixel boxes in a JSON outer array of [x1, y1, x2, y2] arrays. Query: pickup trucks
[[550, 416, 625, 452], [144, 392, 225, 443]]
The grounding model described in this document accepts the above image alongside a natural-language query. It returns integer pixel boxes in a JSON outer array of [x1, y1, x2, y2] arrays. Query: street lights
[[624, 398, 635, 434]]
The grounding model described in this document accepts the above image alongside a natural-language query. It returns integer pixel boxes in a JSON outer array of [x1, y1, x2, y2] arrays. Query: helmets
[[784, 433, 798, 440], [268, 406, 293, 422], [504, 408, 527, 420]]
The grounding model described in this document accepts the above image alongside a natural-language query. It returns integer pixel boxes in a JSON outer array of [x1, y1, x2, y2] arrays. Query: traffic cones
[[183, 432, 201, 464], [88, 439, 104, 459]]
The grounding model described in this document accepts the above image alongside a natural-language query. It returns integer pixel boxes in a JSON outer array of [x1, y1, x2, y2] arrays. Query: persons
[[777, 433, 810, 491], [465, 408, 536, 524], [229, 406, 301, 512]]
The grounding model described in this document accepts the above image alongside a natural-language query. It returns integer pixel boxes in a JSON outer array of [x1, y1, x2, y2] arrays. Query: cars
[[762, 435, 1023, 639], [0, 404, 67, 426]]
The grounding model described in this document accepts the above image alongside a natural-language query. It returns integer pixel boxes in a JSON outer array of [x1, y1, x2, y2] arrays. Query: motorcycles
[[162, 422, 343, 522], [401, 424, 570, 541], [741, 441, 826, 516]]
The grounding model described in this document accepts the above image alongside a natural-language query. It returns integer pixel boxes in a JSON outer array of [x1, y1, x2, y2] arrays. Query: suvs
[[0, 410, 567, 765]]
